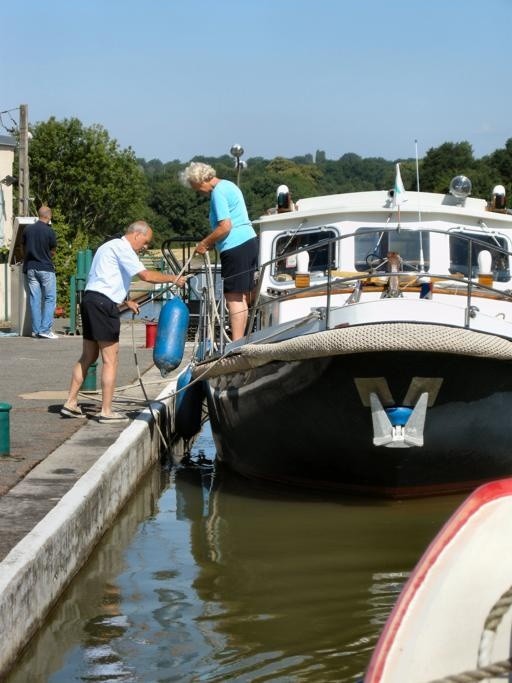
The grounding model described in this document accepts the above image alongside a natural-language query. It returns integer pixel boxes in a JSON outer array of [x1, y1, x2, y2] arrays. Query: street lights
[[227, 144, 248, 188]]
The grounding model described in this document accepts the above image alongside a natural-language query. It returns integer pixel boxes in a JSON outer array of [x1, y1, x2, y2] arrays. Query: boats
[[149, 281, 190, 299], [363, 478, 511, 683], [202, 138, 512, 499]]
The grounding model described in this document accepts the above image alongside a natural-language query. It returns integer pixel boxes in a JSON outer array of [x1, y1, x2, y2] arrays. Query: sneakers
[[61, 403, 87, 419], [98, 413, 130, 423], [32, 332, 58, 340]]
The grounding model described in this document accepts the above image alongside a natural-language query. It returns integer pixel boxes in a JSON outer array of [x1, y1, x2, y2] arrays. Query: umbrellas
[[392, 164, 410, 229]]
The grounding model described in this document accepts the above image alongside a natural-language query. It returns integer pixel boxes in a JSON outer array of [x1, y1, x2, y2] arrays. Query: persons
[[57, 219, 186, 425], [178, 159, 259, 342], [18, 204, 59, 340]]
